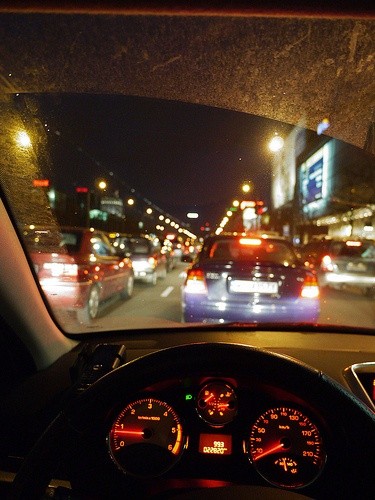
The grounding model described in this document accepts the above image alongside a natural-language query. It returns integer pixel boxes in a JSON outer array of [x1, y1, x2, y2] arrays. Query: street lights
[[267, 127, 295, 243]]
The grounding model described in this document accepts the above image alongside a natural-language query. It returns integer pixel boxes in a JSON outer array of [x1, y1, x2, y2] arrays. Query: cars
[[180, 231, 321, 325], [149, 233, 201, 274], [301, 239, 375, 299], [20, 225, 135, 326], [104, 232, 168, 285]]
